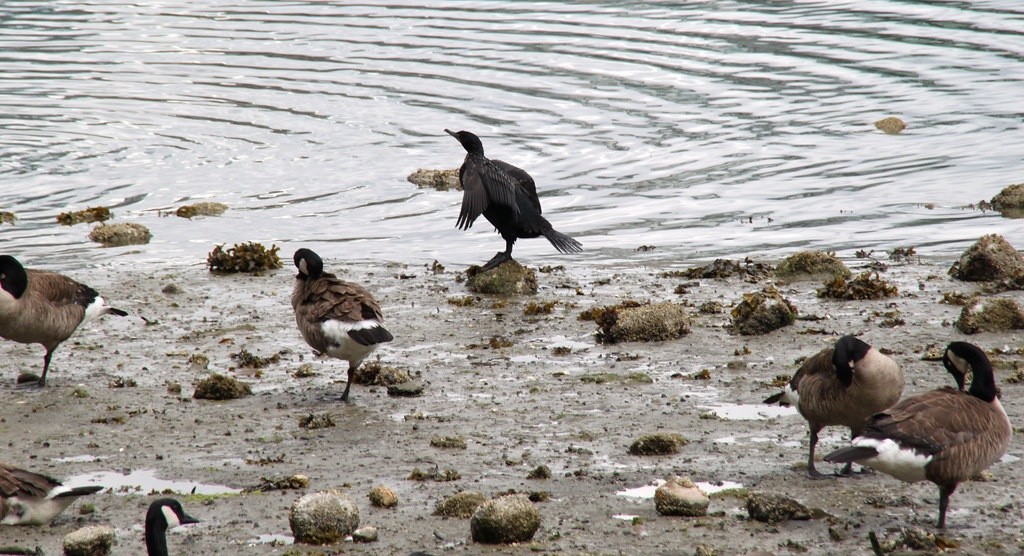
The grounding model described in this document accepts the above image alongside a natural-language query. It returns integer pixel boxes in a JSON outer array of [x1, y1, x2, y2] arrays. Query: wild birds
[[822, 341, 1017, 547], [141, 498, 200, 556], [0, 252, 128, 392], [0, 463, 104, 530], [763, 337, 904, 481], [290, 247, 394, 402], [443, 128, 584, 272]]
